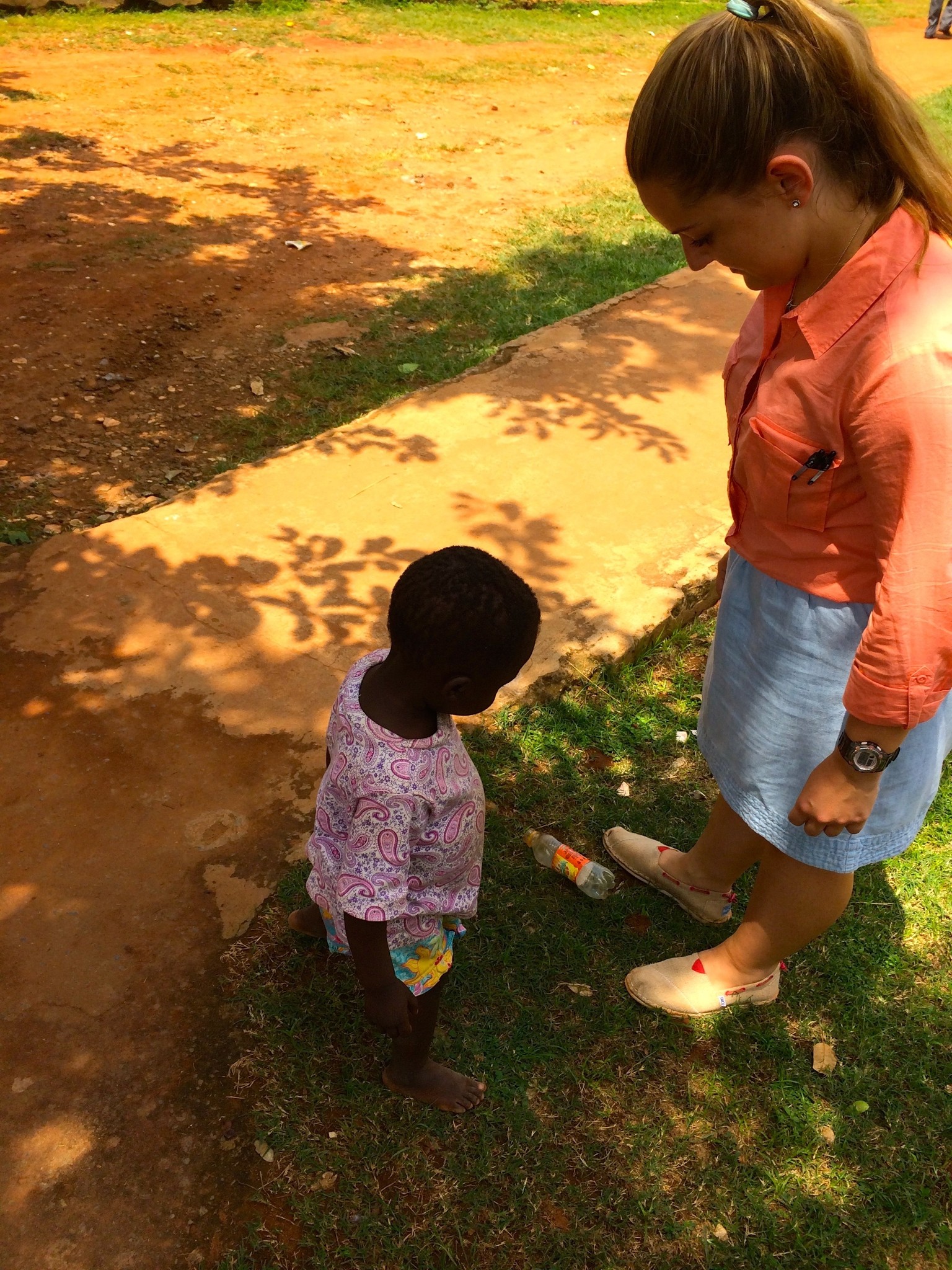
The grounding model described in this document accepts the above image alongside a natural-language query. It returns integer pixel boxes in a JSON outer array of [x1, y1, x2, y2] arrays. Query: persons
[[597, 0, 952, 1019], [307, 546, 540, 1113], [924, 0, 952, 39]]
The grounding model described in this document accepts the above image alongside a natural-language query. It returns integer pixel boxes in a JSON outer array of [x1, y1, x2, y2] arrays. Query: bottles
[[523, 828, 615, 900]]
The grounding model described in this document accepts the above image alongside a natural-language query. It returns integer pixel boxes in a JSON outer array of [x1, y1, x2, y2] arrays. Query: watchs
[[837, 729, 901, 774]]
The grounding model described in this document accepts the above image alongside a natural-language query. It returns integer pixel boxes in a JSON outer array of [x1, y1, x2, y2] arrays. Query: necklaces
[[786, 197, 873, 307]]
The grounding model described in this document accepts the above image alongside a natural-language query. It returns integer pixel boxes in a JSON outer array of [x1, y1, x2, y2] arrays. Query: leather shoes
[[939, 28, 952, 37]]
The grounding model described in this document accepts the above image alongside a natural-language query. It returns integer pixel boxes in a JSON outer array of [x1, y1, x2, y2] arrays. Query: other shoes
[[603, 826, 737, 924], [625, 952, 786, 1019]]
